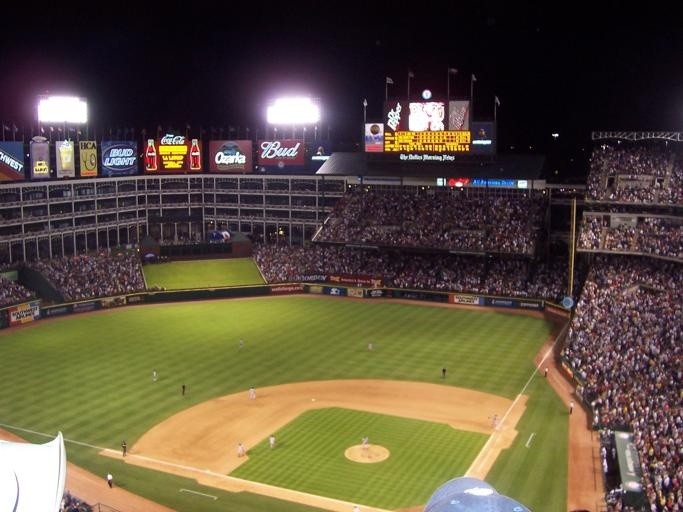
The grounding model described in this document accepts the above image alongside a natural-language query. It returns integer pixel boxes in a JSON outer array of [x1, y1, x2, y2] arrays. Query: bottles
[[189, 139, 201, 170], [145, 139, 157, 171]]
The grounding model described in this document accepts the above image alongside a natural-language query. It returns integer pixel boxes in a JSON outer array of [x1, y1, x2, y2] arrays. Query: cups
[[59, 147, 73, 170]]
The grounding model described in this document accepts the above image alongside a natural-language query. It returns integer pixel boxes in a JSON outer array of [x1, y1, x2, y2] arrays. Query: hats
[[0, 432, 66, 512], [424, 477, 531, 511]]
